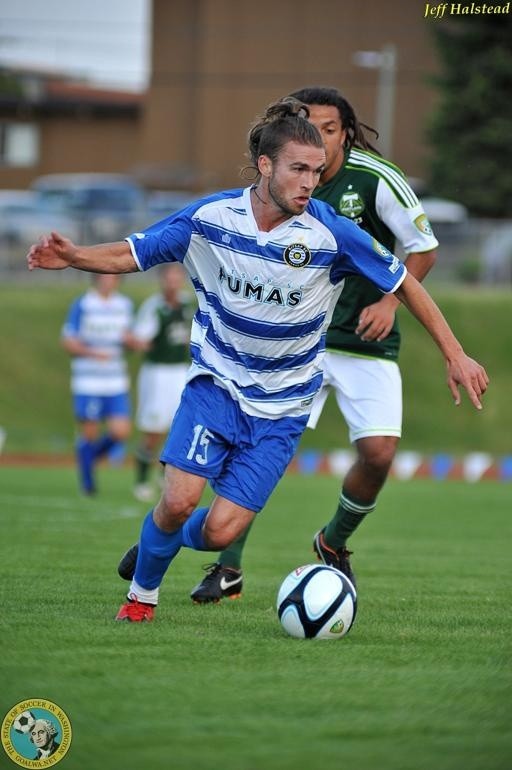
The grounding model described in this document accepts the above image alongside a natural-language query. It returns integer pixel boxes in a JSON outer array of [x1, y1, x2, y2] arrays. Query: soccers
[[277, 563, 357, 637]]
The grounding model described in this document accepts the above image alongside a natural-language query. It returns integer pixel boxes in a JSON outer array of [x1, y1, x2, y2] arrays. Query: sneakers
[[311, 525, 359, 592], [190, 562, 244, 606], [117, 540, 155, 623]]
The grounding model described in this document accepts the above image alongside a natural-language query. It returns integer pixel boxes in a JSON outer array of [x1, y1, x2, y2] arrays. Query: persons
[[58, 270, 137, 501], [133, 263, 196, 506], [189, 87, 440, 602], [24, 94, 489, 624]]
